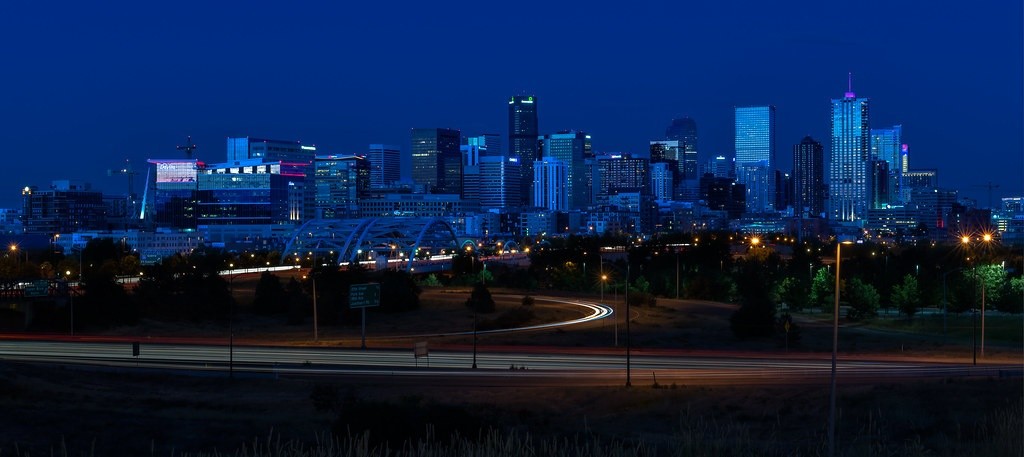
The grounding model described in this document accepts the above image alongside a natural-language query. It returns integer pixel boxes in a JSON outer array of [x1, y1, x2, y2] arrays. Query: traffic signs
[[349, 283, 382, 308]]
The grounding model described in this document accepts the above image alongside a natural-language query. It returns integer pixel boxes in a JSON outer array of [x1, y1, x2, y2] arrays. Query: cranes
[[107, 168, 144, 200], [176, 135, 197, 159]]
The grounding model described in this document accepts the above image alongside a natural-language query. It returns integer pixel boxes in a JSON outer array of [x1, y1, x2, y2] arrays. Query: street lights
[[601, 275, 620, 345], [829, 235, 857, 456], [301, 272, 322, 341], [941, 265, 964, 334], [583, 252, 604, 303], [229, 271, 260, 378], [66, 270, 73, 336], [955, 230, 997, 366]]
